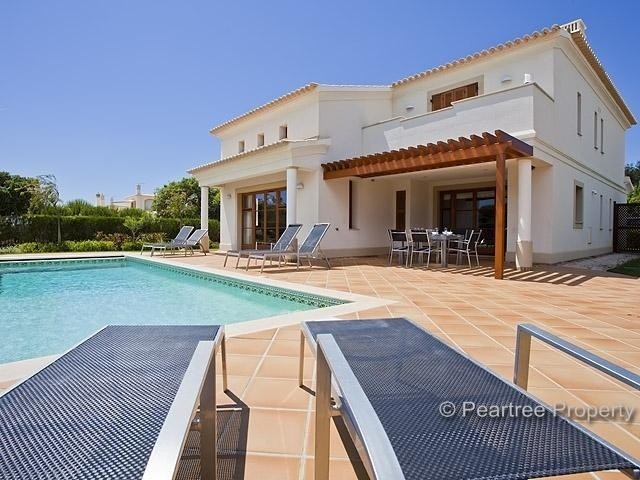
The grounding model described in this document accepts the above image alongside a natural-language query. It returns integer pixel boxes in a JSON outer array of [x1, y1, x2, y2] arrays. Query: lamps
[[226, 194, 231, 199], [296, 183, 304, 190]]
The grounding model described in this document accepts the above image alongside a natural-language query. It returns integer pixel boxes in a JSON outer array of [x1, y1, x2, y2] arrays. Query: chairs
[[295, 310, 640, 479], [150, 228, 210, 257], [1, 322, 227, 480], [222, 221, 304, 271], [383, 226, 488, 271], [141, 224, 196, 256], [243, 222, 335, 273]]
